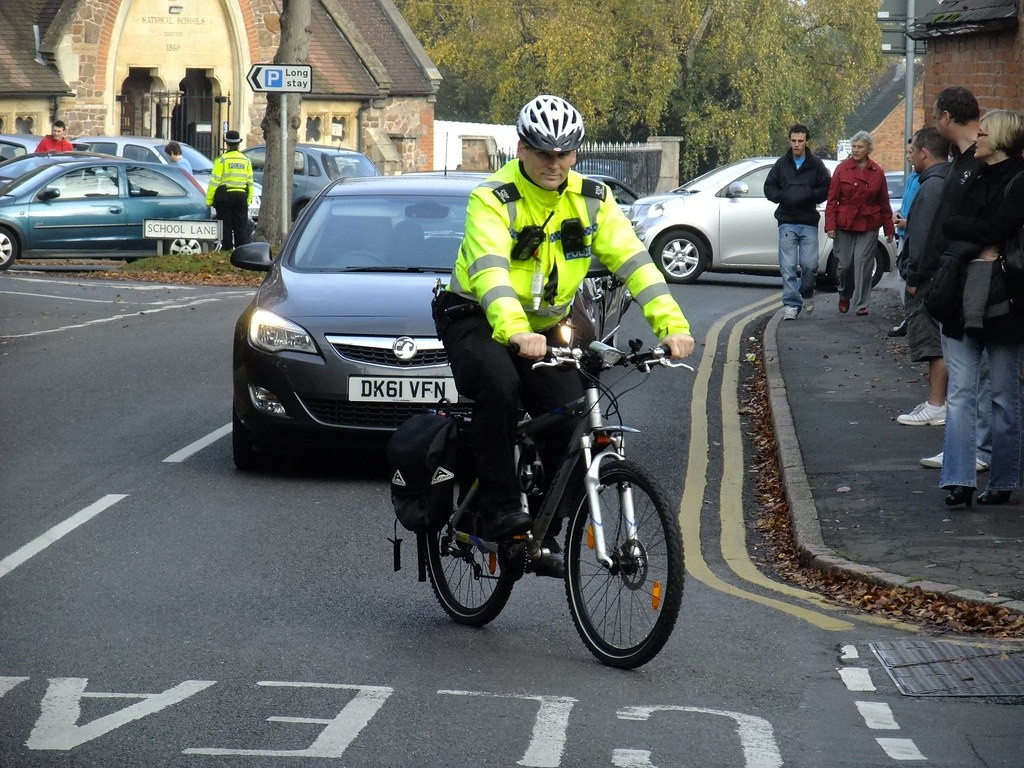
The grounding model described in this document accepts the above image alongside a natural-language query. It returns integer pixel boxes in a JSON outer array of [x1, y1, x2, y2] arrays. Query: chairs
[[341, 165, 354, 177], [311, 216, 394, 268], [0, 147, 16, 162]]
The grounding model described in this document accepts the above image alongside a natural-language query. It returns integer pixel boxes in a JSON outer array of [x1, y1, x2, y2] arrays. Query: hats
[[224, 131, 242, 144]]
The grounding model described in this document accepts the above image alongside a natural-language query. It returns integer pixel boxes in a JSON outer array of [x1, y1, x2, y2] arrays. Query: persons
[[165, 141, 192, 175], [886, 84, 1024, 511], [34, 120, 73, 152], [824, 130, 896, 316], [432, 93, 694, 581], [205, 130, 254, 250], [763, 122, 831, 319]]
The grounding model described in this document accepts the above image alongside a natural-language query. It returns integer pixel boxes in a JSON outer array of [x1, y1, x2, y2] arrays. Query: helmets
[[516, 95, 585, 151]]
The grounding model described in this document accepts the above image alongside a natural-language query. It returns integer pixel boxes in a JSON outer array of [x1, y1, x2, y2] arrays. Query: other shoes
[[856, 306, 867, 316], [839, 299, 850, 313], [887, 320, 907, 337]]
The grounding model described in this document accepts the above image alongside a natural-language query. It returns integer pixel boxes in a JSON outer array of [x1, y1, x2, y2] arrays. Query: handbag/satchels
[[388, 398, 466, 534], [925, 252, 967, 323], [1004, 168, 1024, 271]]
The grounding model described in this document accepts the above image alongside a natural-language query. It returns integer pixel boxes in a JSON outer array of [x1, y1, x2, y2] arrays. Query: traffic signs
[[246, 62, 314, 94]]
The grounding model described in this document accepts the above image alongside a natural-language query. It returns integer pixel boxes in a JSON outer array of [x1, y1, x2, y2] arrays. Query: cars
[[241, 143, 383, 224], [0, 132, 91, 162], [882, 171, 903, 240], [73, 133, 262, 220], [575, 158, 644, 219], [228, 175, 610, 476], [625, 154, 899, 296], [0, 152, 213, 271]]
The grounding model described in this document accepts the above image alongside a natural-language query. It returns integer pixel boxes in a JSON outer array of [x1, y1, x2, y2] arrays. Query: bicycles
[[415, 324, 693, 670]]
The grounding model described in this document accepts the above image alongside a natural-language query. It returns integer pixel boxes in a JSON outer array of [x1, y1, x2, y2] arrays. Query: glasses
[[977, 131, 990, 138], [528, 147, 570, 162]]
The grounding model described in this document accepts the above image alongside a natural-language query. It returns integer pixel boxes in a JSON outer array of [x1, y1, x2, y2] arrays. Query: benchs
[[395, 221, 465, 266]]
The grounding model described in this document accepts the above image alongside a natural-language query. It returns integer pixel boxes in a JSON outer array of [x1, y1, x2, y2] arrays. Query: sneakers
[[484, 513, 533, 542], [897, 397, 948, 425], [803, 298, 814, 314], [784, 305, 800, 320], [973, 457, 991, 471], [536, 538, 566, 577], [919, 452, 944, 469]]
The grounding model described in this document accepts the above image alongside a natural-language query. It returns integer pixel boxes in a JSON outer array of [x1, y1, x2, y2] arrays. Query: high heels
[[977, 488, 1012, 505], [945, 484, 974, 508]]
